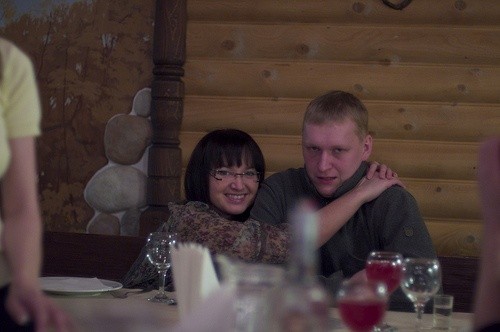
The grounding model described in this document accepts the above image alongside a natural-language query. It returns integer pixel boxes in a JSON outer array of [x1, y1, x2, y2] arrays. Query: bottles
[[266, 200, 333, 332]]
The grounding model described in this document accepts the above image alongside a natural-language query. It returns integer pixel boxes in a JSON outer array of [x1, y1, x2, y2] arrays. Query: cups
[[432, 295, 454, 332]]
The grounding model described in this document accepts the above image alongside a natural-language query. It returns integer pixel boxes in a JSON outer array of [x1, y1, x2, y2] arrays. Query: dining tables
[[34, 276, 477, 331]]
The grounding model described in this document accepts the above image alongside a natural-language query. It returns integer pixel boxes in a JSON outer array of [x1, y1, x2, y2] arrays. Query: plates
[[37, 276, 123, 296]]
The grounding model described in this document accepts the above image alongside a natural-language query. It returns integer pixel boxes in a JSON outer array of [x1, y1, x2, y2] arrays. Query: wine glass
[[147, 232, 180, 303], [337, 278, 388, 332], [399, 257, 442, 331], [363, 252, 406, 332]]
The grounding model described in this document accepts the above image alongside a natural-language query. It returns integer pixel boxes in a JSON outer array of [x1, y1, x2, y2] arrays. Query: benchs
[[38, 232, 480, 316]]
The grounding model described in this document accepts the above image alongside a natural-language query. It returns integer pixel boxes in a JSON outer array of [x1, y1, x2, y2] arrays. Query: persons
[[469, 133, 500, 331], [121, 126, 405, 296], [1, 33, 78, 332], [248, 90, 444, 315]]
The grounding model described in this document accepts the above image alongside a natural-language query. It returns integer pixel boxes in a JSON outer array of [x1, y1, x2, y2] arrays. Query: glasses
[[207, 168, 262, 183]]
